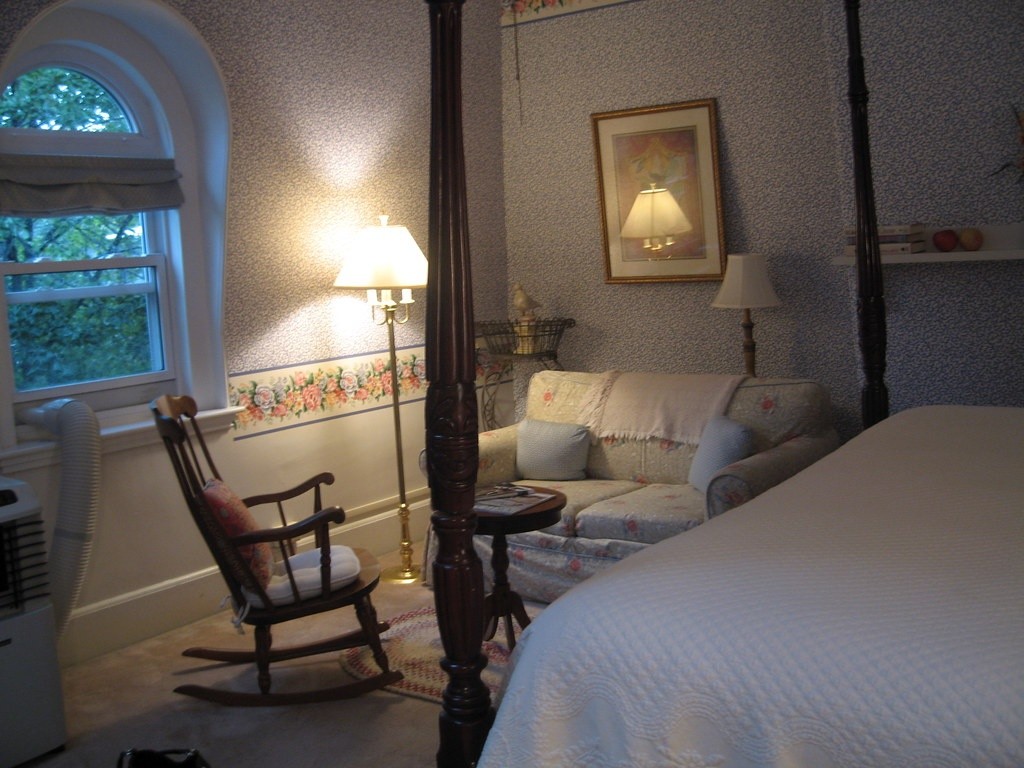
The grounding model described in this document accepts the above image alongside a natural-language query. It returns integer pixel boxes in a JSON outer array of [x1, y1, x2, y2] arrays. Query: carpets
[[338, 605, 549, 707]]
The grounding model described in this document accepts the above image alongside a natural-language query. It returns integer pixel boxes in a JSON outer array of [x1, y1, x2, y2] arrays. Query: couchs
[[418, 370, 840, 604]]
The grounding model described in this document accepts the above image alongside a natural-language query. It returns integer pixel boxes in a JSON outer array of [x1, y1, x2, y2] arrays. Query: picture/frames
[[589, 97, 727, 285]]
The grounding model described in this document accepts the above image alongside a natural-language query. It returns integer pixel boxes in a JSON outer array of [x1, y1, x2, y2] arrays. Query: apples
[[959, 228, 983, 250], [933, 229, 958, 251]]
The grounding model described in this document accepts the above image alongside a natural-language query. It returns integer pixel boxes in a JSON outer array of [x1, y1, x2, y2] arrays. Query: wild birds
[[512, 284, 544, 316]]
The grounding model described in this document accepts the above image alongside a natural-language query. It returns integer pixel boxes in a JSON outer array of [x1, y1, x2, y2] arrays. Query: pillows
[[688, 415, 751, 493], [516, 419, 590, 481], [202, 479, 276, 593]]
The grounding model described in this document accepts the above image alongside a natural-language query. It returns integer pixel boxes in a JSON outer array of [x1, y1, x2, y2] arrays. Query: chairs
[[149, 394, 405, 707]]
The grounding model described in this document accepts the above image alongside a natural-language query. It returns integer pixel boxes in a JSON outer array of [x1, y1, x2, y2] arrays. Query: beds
[[464, 406, 1024, 768]]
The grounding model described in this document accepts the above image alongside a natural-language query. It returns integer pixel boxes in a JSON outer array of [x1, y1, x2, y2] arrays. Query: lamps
[[620, 182, 693, 259], [333, 213, 429, 586], [709, 254, 782, 377]]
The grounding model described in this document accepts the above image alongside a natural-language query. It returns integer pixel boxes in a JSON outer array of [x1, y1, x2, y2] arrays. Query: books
[[475, 480, 558, 516], [844, 222, 927, 256]]
